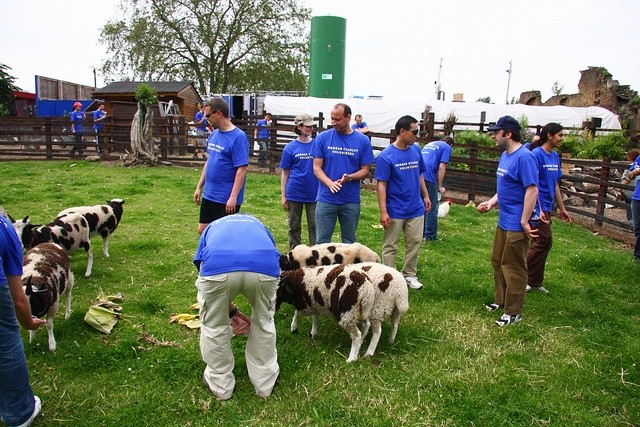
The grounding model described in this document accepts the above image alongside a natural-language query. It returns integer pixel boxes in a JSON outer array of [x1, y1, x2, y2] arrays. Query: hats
[[294, 113, 317, 126], [487, 116, 521, 131], [73, 101, 83, 107]]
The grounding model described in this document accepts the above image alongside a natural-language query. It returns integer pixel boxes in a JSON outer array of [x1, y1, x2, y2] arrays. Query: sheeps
[[275, 265, 375, 364], [280, 242, 381, 269], [8, 213, 93, 277], [353, 260, 408, 346], [59, 199, 126, 258], [22, 243, 75, 354]]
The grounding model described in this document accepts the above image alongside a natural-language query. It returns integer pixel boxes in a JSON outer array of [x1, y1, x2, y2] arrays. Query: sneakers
[[404, 275, 423, 289], [11, 396, 42, 427], [495, 312, 522, 327], [538, 286, 549, 294], [486, 302, 504, 311], [526, 284, 532, 291]]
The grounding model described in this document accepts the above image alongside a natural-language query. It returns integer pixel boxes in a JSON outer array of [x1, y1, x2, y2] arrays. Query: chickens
[[437, 198, 453, 218]]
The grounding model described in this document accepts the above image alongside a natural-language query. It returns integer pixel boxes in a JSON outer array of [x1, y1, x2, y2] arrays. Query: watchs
[[229, 307, 238, 317]]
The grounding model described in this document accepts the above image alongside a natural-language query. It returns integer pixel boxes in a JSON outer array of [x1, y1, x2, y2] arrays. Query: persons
[[525, 123, 571, 293], [193, 98, 249, 233], [421, 136, 455, 241], [70, 102, 89, 156], [374, 115, 431, 289], [192, 214, 280, 399], [254, 112, 272, 167], [620, 148, 640, 233], [195, 101, 212, 159], [628, 153, 640, 262], [281, 113, 319, 250], [524, 135, 539, 149], [476, 116, 540, 328], [310, 103, 375, 243], [0, 216, 47, 426], [351, 114, 371, 142], [94, 102, 108, 155]]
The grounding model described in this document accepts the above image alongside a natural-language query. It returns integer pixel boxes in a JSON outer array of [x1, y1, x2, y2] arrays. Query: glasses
[[404, 129, 418, 135], [205, 110, 217, 118]]
